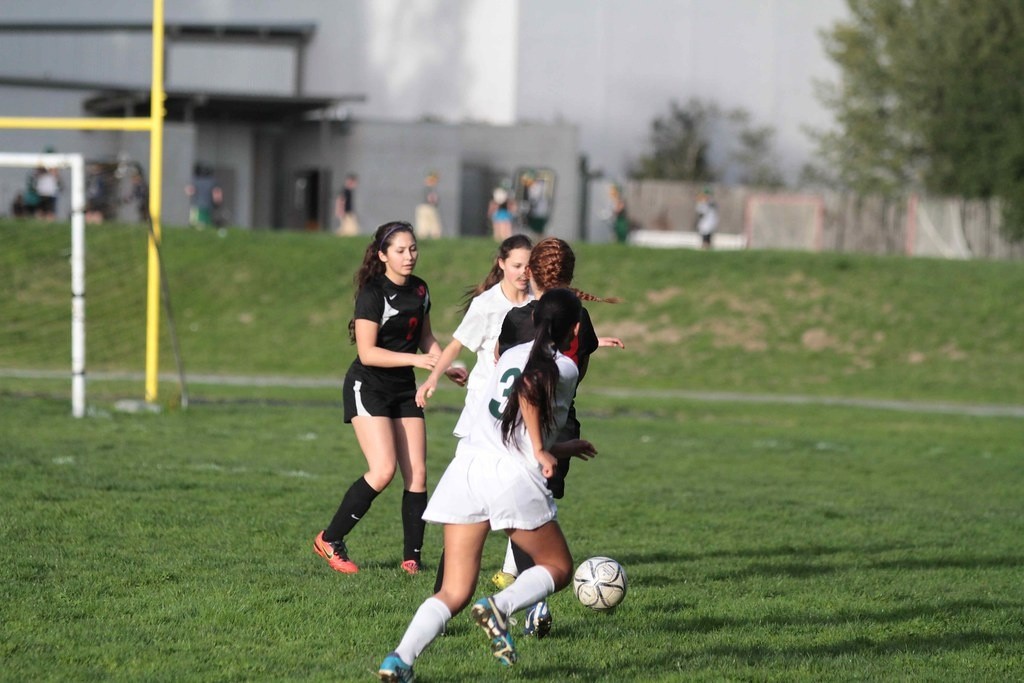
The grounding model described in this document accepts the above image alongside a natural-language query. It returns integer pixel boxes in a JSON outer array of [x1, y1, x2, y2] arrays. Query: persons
[[332, 172, 361, 235], [697, 186, 717, 248], [488, 171, 551, 242], [10, 147, 226, 234], [377, 288, 597, 683], [414, 234, 624, 591], [312, 219, 468, 576], [611, 184, 629, 244], [493, 238, 599, 638], [413, 173, 442, 238]]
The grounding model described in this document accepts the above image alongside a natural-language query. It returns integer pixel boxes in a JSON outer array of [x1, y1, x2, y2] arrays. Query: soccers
[[571, 556, 629, 613]]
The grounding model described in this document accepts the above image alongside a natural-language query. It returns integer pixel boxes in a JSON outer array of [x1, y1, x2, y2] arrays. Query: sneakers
[[472, 596, 518, 666], [312, 526, 358, 573], [491, 570, 516, 590], [524, 602, 551, 637], [402, 559, 419, 574], [379, 653, 415, 683]]
